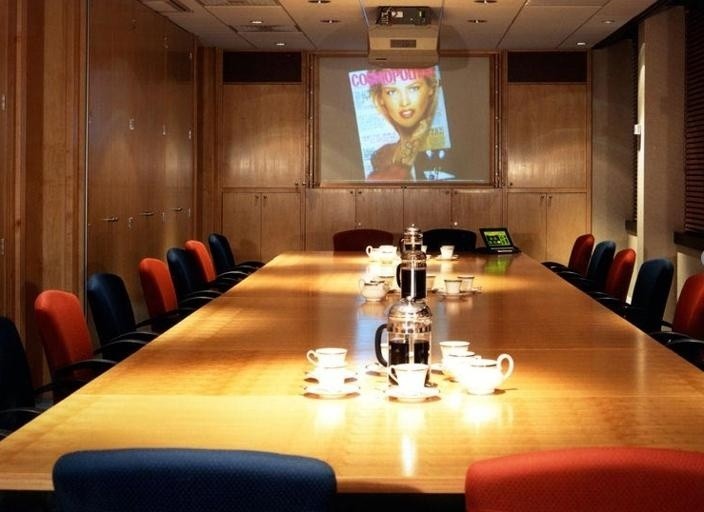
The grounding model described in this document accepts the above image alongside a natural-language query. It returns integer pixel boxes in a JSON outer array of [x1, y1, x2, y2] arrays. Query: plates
[[440, 292, 466, 300], [426, 287, 440, 293], [459, 288, 479, 292]]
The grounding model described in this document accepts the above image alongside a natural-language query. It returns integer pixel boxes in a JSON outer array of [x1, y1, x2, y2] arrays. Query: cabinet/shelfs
[[506, 191, 588, 269], [220, 187, 300, 264], [403, 188, 503, 249], [305, 186, 403, 251]]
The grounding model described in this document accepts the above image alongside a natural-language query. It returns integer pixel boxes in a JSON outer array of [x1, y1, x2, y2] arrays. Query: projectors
[[367, 24, 440, 68]]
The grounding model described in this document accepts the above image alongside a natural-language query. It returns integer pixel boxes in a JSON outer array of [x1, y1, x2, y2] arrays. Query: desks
[[1, 250, 704, 497]]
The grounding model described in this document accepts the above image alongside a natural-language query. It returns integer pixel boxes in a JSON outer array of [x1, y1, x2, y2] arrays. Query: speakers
[[509, 52, 587, 81], [223, 52, 301, 82]]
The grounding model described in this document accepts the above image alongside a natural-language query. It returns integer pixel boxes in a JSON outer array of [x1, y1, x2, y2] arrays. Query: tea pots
[[396, 248, 429, 301], [454, 352, 514, 395], [375, 295, 433, 385], [398, 223, 424, 255], [357, 278, 391, 302]]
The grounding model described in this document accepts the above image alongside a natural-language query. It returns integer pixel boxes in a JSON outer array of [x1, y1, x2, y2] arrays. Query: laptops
[[480, 228, 521, 252]]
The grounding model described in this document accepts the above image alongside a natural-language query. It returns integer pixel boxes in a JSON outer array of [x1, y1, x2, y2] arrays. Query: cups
[[301, 339, 484, 403], [426, 275, 436, 289], [366, 244, 397, 264], [421, 244, 461, 261], [369, 275, 397, 293], [458, 276, 475, 290], [445, 279, 462, 293]]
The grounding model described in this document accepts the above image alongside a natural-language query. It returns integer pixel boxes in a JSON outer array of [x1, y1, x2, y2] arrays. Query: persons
[[368, 65, 445, 182]]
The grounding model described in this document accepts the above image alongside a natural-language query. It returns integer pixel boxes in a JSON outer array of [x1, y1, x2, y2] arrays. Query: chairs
[[331, 228, 394, 252], [464, 447, 704, 512], [47, 446, 338, 512], [422, 228, 477, 254], [540, 231, 704, 367]]
